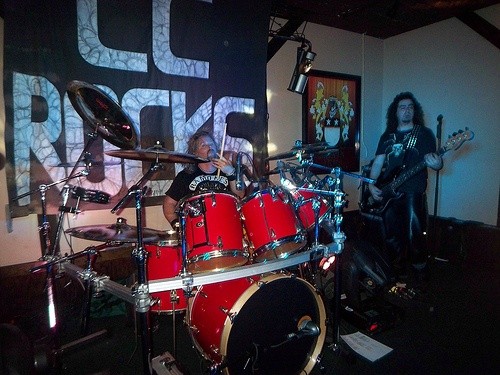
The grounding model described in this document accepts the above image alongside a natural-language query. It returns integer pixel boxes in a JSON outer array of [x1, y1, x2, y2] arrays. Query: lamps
[[288, 47, 318, 95]]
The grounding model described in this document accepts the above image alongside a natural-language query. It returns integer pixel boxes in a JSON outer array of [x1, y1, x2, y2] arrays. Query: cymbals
[[268, 146, 337, 160], [286, 149, 339, 161], [101, 149, 210, 164], [71, 186, 111, 205], [66, 79, 139, 150], [65, 223, 170, 243], [266, 165, 295, 175]]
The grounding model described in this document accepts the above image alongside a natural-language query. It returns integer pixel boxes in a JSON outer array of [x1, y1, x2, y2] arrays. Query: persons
[[162, 130, 245, 231], [368, 91, 443, 277]]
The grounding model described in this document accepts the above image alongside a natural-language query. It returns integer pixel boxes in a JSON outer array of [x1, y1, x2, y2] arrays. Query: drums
[[237, 187, 307, 263], [292, 181, 333, 232], [145, 239, 190, 313], [186, 269, 326, 375], [182, 191, 249, 271]]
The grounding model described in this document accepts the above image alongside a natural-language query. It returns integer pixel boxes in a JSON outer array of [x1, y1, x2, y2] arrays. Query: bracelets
[[227, 173, 237, 181], [171, 218, 179, 230]]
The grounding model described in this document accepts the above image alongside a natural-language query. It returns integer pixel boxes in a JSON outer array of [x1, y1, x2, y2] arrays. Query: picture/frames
[[303, 69, 361, 175]]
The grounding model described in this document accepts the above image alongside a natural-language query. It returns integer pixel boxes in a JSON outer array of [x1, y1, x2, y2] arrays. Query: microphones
[[296, 319, 320, 340], [235, 152, 243, 191], [286, 163, 301, 185]]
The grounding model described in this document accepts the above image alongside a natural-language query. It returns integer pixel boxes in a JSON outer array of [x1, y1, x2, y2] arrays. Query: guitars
[[358, 126, 475, 219]]
